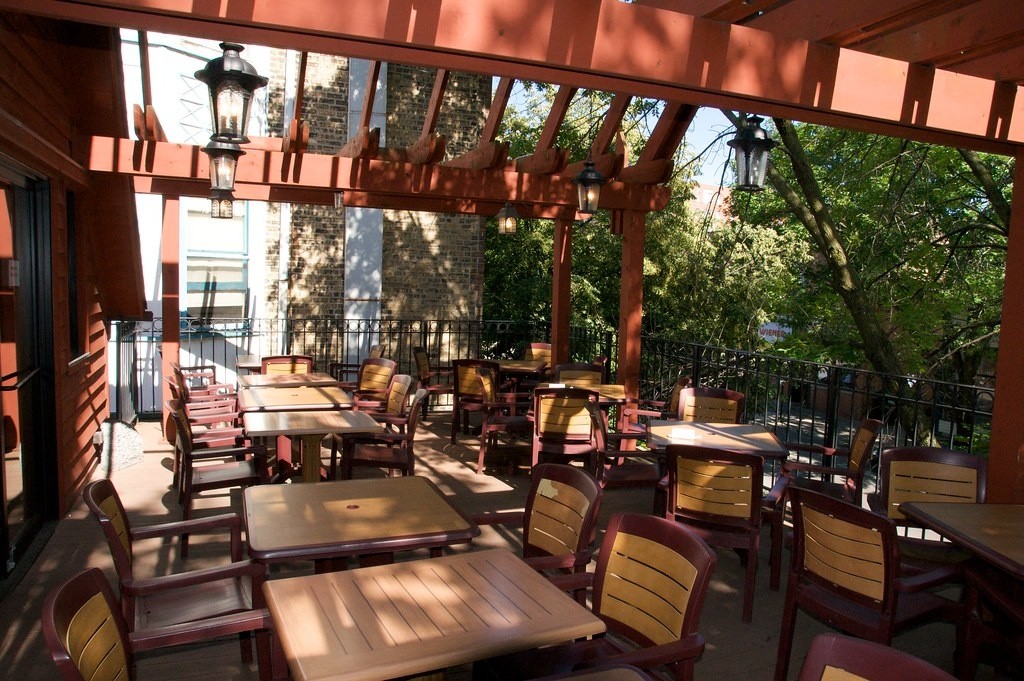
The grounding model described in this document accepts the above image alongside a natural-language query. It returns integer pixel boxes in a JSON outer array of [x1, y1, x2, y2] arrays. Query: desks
[[237, 386, 357, 411], [645, 420, 790, 461], [477, 358, 548, 417], [261, 545, 609, 681], [240, 475, 482, 577], [529, 382, 626, 432], [243, 410, 385, 483], [237, 371, 339, 387], [898, 501, 1024, 681]]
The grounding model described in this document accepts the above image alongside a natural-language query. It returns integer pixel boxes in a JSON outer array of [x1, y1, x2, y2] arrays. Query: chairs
[[39, 340, 1024, 681]]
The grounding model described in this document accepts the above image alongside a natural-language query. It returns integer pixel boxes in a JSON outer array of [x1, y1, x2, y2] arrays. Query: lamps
[[726, 113, 781, 190], [193, 42, 269, 143], [572, 157, 607, 213], [200, 140, 247, 219], [494, 202, 519, 234]]
